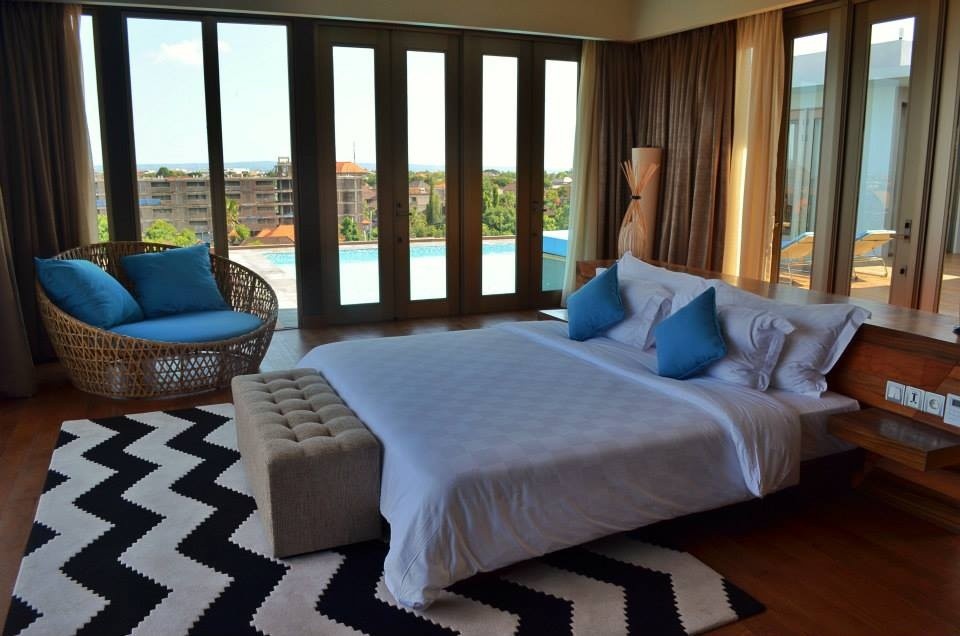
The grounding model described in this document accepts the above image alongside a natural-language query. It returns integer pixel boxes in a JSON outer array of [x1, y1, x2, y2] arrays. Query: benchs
[[230, 368, 381, 557]]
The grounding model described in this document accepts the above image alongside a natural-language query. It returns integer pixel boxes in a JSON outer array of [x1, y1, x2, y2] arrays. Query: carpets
[[3, 402, 766, 636]]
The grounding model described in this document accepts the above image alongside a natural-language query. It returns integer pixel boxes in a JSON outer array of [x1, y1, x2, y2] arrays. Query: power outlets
[[923, 391, 945, 417], [904, 385, 925, 411]]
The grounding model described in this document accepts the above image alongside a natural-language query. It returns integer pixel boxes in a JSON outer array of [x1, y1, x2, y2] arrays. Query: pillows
[[708, 280, 872, 399], [567, 263, 627, 341], [620, 250, 705, 293], [594, 267, 671, 350], [672, 291, 795, 392], [121, 243, 230, 317], [35, 257, 143, 329], [656, 286, 727, 380]]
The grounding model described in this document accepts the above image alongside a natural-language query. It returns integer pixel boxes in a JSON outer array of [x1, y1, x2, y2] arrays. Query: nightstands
[[538, 309, 567, 323], [827, 407, 960, 471]]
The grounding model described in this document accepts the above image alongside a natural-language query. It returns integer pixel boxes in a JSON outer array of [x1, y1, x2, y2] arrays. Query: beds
[[293, 261, 959, 611]]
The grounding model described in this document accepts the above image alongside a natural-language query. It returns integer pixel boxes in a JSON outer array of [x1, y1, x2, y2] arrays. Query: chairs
[[35, 241, 278, 398], [779, 231, 814, 282], [851, 231, 896, 277]]
